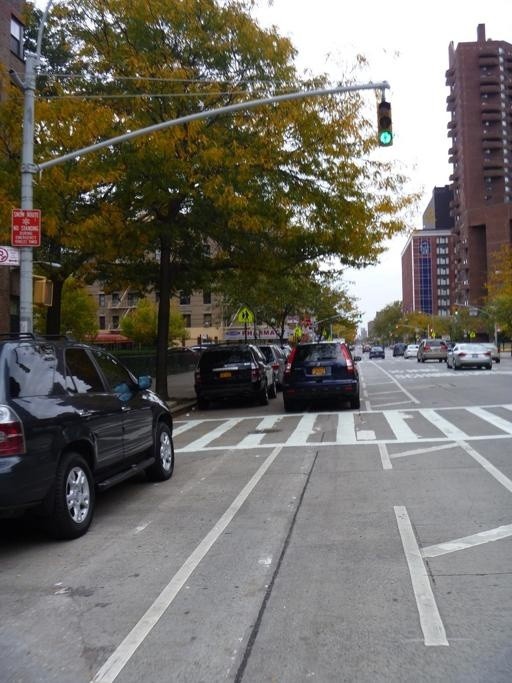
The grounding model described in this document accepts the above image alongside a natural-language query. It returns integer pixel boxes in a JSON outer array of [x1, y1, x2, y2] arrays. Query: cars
[[447, 342, 456, 350], [363, 338, 419, 360], [447, 342, 493, 371], [346, 343, 354, 349], [480, 344, 500, 364], [191, 344, 207, 355], [167, 346, 200, 364]]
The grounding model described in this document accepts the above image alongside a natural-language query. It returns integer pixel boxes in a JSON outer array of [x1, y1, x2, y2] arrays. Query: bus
[[74, 329, 177, 351]]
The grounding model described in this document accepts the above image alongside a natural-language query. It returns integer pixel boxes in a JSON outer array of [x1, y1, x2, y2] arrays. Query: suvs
[[417, 338, 448, 363], [194, 341, 275, 409], [282, 336, 359, 413], [0, 333, 177, 538], [258, 341, 291, 387]]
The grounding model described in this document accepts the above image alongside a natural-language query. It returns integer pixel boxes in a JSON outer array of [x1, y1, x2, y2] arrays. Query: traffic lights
[[377, 101, 395, 146]]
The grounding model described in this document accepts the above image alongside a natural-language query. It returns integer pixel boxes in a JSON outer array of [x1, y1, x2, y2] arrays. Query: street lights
[[418, 310, 432, 317], [395, 325, 419, 340], [454, 303, 497, 342]]
